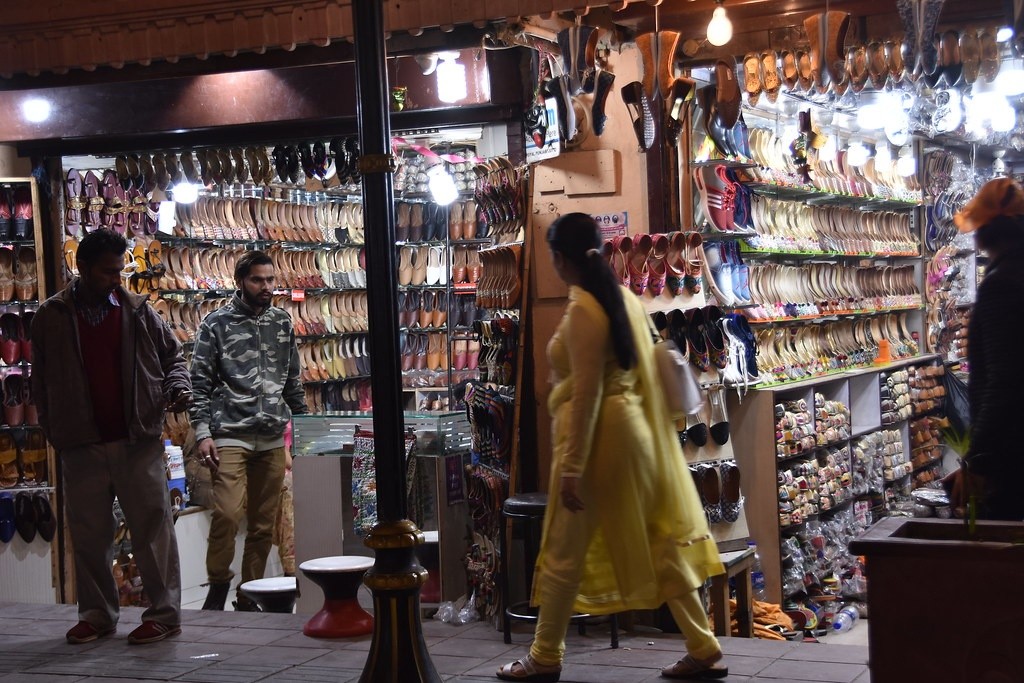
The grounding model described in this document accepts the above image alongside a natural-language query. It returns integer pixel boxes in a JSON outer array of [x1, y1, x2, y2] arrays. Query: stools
[[411, 530, 441, 603], [500, 494, 618, 650], [298, 556, 375, 639], [240, 577, 296, 613]]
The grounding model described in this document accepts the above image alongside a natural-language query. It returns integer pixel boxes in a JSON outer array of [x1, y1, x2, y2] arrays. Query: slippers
[[496, 653, 560, 683], [661, 654, 728, 679]]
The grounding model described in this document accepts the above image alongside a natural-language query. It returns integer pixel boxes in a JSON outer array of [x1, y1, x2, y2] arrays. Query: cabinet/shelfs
[[693, 100, 945, 602], [0, 178, 58, 494]]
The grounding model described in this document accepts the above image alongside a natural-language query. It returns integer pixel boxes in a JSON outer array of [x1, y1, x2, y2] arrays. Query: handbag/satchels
[[632, 294, 702, 417]]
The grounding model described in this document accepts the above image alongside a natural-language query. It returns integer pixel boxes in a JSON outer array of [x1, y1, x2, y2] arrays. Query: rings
[[203, 458, 206, 461], [200, 458, 203, 462], [566, 496, 575, 502], [206, 454, 211, 460]]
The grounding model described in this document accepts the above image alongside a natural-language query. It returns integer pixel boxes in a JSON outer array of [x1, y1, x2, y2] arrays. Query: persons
[[942, 177, 1024, 521], [31, 227, 195, 643], [187, 250, 312, 609], [495, 211, 729, 683], [181, 415, 297, 580]]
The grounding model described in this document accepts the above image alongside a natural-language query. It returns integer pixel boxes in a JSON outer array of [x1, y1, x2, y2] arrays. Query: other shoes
[[0, 138, 525, 612], [128, 621, 181, 643], [66, 621, 116, 643], [548, 11, 1024, 643]]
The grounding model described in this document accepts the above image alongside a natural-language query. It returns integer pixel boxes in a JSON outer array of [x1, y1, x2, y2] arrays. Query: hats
[[953, 178, 1024, 234]]
